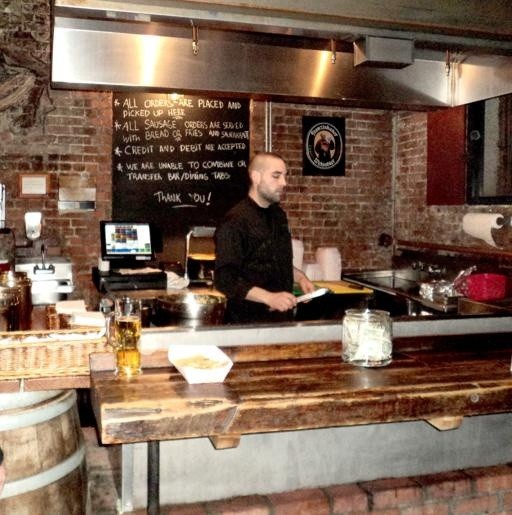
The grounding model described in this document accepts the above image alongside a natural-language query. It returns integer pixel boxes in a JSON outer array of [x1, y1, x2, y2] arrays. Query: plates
[[167, 345, 233, 384]]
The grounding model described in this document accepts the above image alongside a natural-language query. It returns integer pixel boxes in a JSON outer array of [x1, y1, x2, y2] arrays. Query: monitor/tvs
[[99, 218, 159, 264]]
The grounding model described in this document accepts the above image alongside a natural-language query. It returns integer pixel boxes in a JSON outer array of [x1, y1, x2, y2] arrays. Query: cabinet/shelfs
[[425, 93, 512, 206]]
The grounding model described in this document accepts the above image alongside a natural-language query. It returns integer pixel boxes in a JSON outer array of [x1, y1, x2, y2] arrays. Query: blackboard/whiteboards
[[112, 92, 251, 238]]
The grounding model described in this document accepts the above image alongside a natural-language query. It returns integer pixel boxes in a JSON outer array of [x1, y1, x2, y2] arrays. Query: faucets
[[31, 244, 56, 273], [410, 259, 446, 277]]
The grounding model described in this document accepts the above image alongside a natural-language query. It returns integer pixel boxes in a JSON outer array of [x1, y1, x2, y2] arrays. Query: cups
[[342, 308, 393, 368], [105, 297, 142, 351]]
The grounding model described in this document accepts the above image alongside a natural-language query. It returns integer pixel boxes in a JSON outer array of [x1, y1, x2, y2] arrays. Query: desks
[[90, 348, 512, 515]]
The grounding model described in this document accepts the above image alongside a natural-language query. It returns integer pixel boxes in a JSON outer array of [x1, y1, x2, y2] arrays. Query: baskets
[[2, 330, 116, 379]]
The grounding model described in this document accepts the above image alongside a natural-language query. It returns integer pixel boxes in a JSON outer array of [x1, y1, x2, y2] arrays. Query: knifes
[[269, 287, 329, 312]]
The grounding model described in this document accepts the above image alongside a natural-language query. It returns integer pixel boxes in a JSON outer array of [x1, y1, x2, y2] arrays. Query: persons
[[213, 150, 315, 324], [315, 132, 335, 163]]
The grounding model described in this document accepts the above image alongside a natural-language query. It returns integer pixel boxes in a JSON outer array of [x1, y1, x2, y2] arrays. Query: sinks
[[18, 263, 72, 282], [348, 270, 422, 292]]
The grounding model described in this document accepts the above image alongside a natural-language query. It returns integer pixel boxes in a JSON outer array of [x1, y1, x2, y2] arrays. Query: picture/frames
[[19, 172, 51, 197]]
